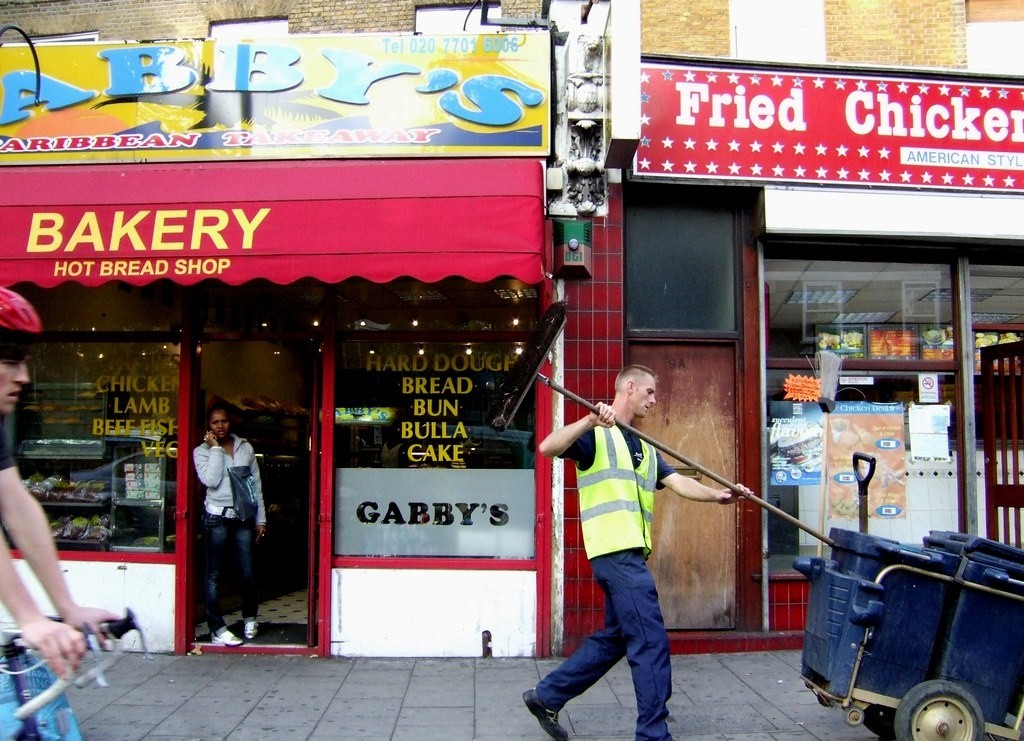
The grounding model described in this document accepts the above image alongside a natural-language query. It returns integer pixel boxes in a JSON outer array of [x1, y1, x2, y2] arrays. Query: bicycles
[[0, 608, 154, 741]]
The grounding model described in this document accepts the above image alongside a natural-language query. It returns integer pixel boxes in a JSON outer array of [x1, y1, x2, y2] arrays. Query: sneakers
[[212, 625, 243, 646], [522, 688, 568, 741], [243, 617, 258, 639]]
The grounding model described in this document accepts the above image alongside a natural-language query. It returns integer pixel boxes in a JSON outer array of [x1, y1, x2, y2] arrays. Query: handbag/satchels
[[227, 465, 258, 522]]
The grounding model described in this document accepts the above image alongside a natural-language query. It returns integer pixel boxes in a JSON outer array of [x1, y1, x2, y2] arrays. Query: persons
[[192, 401, 267, 647], [0, 286, 124, 678], [522, 365, 753, 741]]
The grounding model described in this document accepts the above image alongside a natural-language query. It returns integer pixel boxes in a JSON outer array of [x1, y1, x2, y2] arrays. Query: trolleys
[[798, 450, 1024, 741]]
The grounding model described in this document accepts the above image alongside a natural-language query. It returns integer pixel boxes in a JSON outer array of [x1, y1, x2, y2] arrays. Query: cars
[[68, 445, 177, 508]]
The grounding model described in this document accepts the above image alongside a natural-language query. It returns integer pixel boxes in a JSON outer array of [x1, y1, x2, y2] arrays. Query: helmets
[[0, 286, 44, 335]]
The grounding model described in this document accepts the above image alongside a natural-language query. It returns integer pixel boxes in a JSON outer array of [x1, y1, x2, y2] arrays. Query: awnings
[[2, 159, 553, 289]]
